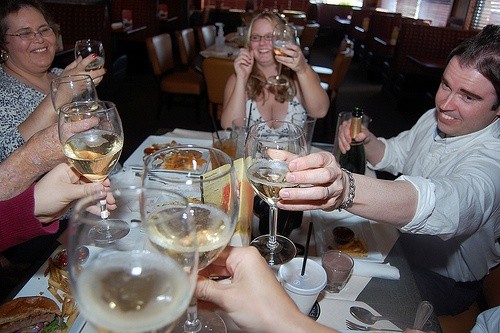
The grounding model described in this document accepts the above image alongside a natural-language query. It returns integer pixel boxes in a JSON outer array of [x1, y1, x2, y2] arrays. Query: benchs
[[42, 0, 188, 93]]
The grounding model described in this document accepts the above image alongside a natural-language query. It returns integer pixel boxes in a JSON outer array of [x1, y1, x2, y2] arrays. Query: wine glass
[[57, 101, 131, 243], [267, 25, 295, 86], [244, 120, 307, 266], [139, 145, 239, 333]]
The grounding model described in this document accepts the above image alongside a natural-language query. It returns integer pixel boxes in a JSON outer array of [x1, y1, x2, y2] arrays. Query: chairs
[[201, 55, 235, 115], [147, 20, 226, 127], [288, 14, 354, 142]]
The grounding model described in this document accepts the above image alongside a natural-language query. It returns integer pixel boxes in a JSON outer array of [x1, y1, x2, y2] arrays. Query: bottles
[[339, 107, 366, 175]]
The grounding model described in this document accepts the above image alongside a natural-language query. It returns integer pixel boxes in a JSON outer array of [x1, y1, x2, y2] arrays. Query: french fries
[[45, 257, 81, 327]]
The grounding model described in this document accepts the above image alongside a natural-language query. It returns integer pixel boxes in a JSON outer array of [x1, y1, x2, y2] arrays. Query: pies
[[0, 296, 62, 333]]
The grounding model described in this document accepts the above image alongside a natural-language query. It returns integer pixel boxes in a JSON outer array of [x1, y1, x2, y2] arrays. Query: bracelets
[[336, 168, 356, 212]]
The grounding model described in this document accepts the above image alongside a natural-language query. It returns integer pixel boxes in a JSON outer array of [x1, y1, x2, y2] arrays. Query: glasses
[[2, 27, 54, 40], [249, 34, 280, 41]]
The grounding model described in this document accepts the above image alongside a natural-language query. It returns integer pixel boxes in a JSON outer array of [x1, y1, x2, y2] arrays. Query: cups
[[212, 131, 238, 170], [322, 250, 354, 293], [237, 27, 248, 39], [75, 39, 104, 72], [231, 117, 256, 159], [49, 75, 98, 122], [65, 186, 199, 333], [277, 257, 327, 316], [292, 112, 316, 154], [333, 113, 369, 162]]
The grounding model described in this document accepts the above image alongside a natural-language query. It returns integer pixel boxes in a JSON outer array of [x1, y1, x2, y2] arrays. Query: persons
[[188, 246, 500, 333], [220, 12, 331, 157], [0, 0, 119, 306], [266, 24, 500, 315]]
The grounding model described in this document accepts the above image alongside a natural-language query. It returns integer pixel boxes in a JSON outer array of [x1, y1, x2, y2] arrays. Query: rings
[[323, 185, 329, 200]]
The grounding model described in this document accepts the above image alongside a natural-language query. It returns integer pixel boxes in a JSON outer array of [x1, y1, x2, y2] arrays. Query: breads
[[164, 150, 208, 170], [337, 240, 368, 257]]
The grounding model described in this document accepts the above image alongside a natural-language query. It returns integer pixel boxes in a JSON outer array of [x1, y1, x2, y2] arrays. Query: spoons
[[350, 306, 414, 325]]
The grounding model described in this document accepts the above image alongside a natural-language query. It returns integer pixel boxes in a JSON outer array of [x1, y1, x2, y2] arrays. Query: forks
[[346, 320, 402, 333]]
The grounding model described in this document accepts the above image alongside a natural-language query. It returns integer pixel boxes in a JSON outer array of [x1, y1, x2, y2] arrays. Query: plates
[[311, 209, 384, 263], [123, 135, 213, 178]]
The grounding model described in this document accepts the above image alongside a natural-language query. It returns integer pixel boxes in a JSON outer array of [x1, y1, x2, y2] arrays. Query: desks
[[11, 128, 442, 333], [199, 27, 249, 121]]
